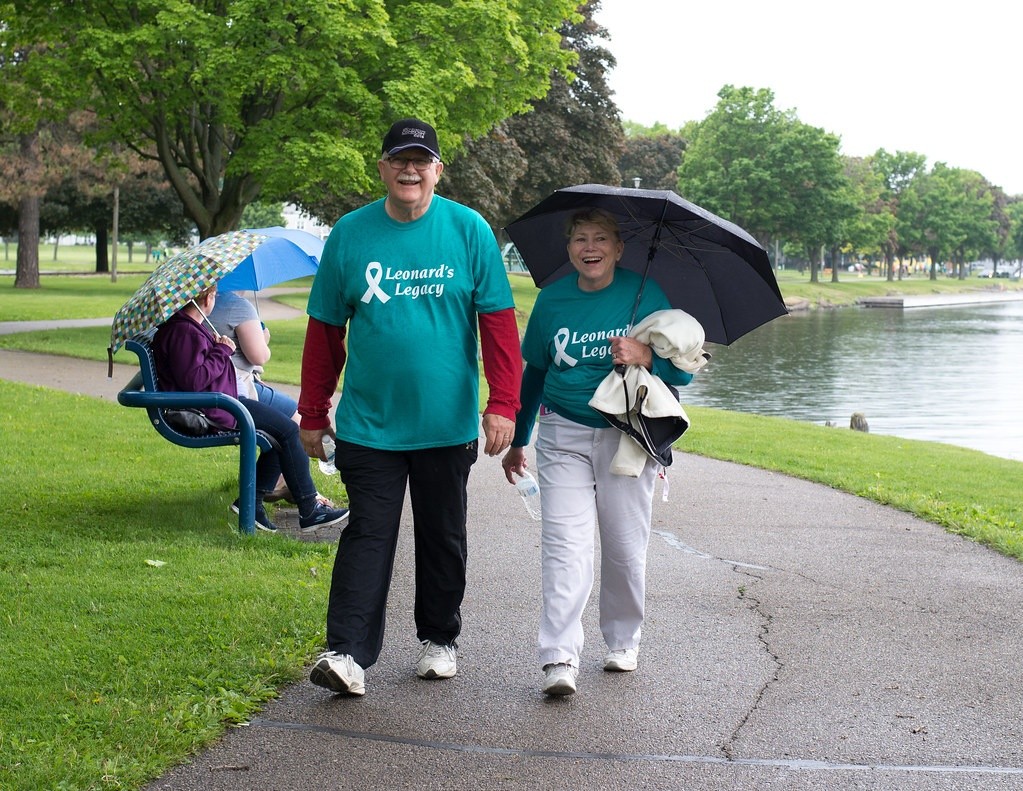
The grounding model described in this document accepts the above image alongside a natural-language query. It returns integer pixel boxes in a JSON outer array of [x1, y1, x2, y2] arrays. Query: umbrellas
[[503, 182, 789, 433], [107, 230, 272, 378], [216, 226, 326, 331]]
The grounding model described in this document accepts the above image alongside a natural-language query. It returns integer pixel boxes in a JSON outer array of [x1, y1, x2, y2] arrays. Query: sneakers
[[310, 651, 365, 695], [412, 639, 457, 679], [604, 647, 638, 671], [232, 497, 278, 533], [299, 501, 351, 533], [542, 663, 577, 695]]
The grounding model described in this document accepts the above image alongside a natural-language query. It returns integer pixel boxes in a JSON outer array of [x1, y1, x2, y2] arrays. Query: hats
[[382, 119, 440, 160]]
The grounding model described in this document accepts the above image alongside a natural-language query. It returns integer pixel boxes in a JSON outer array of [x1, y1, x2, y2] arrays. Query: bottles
[[318, 435, 337, 476], [516, 469, 542, 520]]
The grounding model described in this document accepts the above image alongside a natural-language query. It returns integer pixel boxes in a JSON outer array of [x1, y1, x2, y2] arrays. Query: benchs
[[117, 327, 273, 537]]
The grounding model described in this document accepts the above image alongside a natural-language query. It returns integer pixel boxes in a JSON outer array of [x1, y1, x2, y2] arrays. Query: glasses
[[385, 157, 436, 170]]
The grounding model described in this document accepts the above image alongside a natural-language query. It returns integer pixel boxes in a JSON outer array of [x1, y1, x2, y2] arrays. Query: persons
[[501, 206, 695, 693], [155, 281, 350, 533], [296, 119, 523, 694]]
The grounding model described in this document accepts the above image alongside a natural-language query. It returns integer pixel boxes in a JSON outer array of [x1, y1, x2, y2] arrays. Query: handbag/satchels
[[163, 408, 241, 437]]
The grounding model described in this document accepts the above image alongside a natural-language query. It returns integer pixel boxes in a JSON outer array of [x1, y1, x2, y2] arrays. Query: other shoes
[[264, 486, 295, 504], [316, 495, 335, 507]]
